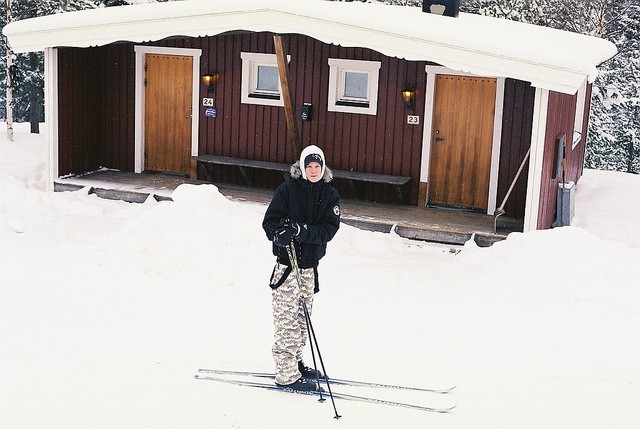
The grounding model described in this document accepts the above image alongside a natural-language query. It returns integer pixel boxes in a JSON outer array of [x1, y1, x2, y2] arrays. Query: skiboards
[[195, 368, 459, 413]]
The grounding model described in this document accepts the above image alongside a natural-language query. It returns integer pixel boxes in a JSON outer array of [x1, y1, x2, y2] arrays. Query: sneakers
[[275, 379, 317, 392], [298, 360, 321, 379]]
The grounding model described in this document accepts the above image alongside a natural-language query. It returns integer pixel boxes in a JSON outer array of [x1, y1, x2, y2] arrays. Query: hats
[[305, 154, 322, 168]]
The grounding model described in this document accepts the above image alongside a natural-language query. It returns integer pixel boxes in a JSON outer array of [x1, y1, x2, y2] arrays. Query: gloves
[[268, 216, 298, 247]]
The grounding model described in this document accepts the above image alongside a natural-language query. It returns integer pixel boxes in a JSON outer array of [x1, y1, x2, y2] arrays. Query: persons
[[261, 143, 341, 396]]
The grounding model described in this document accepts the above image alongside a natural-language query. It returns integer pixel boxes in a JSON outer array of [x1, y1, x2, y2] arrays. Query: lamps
[[400, 88, 416, 109], [202, 73, 215, 93]]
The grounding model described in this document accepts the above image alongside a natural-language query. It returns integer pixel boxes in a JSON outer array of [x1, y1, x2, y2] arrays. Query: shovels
[[494, 146, 530, 234]]
